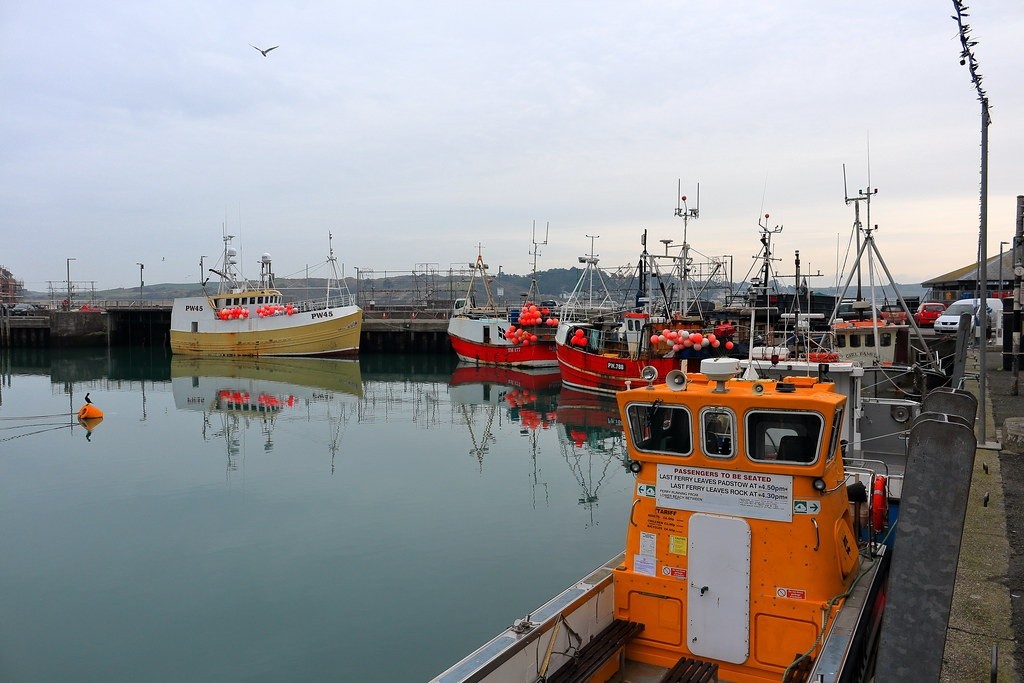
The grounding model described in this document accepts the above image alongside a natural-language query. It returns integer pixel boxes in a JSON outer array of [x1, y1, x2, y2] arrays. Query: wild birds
[[86, 430, 91, 442], [162, 257, 165, 261], [248, 43, 280, 57], [85, 392, 92, 403]]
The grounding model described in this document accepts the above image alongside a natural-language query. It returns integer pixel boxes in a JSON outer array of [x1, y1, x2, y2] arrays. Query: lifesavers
[[810, 352, 839, 363], [872, 475, 886, 534]]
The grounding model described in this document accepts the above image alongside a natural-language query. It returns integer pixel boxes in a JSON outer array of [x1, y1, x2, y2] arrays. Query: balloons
[[218, 308, 250, 320], [519, 410, 557, 430], [518, 302, 558, 326], [257, 304, 298, 318], [571, 429, 587, 448], [571, 329, 587, 347], [506, 389, 536, 407], [505, 326, 536, 346], [651, 329, 733, 351], [219, 389, 300, 406]]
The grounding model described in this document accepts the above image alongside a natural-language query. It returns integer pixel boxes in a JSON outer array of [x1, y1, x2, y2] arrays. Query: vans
[[933, 297, 1003, 335]]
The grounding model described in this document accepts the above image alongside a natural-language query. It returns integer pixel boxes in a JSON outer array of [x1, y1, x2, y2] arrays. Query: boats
[[0, 132, 958, 682]]
